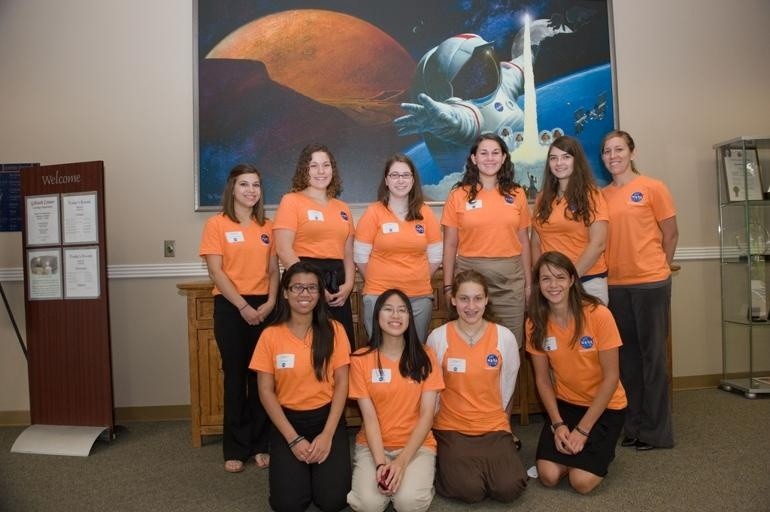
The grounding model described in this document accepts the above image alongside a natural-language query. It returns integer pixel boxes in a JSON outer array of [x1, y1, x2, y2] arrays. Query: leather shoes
[[637, 435, 676, 452], [620, 435, 640, 447]]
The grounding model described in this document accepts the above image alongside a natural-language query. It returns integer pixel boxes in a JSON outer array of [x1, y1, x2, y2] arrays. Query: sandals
[[253, 452, 272, 469], [223, 460, 246, 474]]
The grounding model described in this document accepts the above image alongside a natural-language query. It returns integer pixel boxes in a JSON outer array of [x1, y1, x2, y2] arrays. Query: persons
[[496, 126, 565, 152], [531, 137, 611, 308], [201, 163, 279, 473], [271, 142, 356, 352], [442, 134, 533, 453], [427, 268, 530, 502], [522, 248, 630, 495], [352, 154, 443, 342], [35, 259, 43, 269], [44, 261, 51, 274], [599, 130, 682, 453], [245, 261, 351, 512], [345, 286, 445, 512], [394, 31, 535, 175]]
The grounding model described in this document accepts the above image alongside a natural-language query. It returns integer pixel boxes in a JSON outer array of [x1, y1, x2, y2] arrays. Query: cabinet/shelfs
[[712, 129, 770, 399], [181, 260, 681, 444]]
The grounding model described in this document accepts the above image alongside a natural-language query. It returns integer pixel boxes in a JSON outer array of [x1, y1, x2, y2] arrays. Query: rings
[[557, 447, 560, 452], [558, 444, 562, 449]]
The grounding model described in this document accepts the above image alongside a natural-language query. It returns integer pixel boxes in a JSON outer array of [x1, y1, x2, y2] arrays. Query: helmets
[[426, 31, 502, 100]]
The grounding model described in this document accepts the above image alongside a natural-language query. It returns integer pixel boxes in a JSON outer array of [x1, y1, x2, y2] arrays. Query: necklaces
[[389, 205, 410, 217], [293, 327, 310, 350], [611, 180, 626, 187], [556, 193, 564, 205], [482, 183, 495, 192], [458, 321, 484, 349]]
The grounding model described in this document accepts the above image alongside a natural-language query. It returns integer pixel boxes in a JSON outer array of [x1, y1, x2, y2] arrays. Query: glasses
[[287, 283, 320, 295], [386, 171, 413, 180]]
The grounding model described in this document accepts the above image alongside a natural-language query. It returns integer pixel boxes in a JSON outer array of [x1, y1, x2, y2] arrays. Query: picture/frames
[[188, 0, 625, 218]]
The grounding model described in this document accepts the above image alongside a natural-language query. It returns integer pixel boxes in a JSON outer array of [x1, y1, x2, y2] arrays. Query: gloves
[[392, 92, 463, 139]]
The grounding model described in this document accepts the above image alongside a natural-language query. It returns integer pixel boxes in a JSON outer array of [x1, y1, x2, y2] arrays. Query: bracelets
[[288, 435, 305, 447], [376, 463, 385, 470], [550, 422, 566, 434], [238, 302, 249, 313], [575, 425, 592, 437]]
